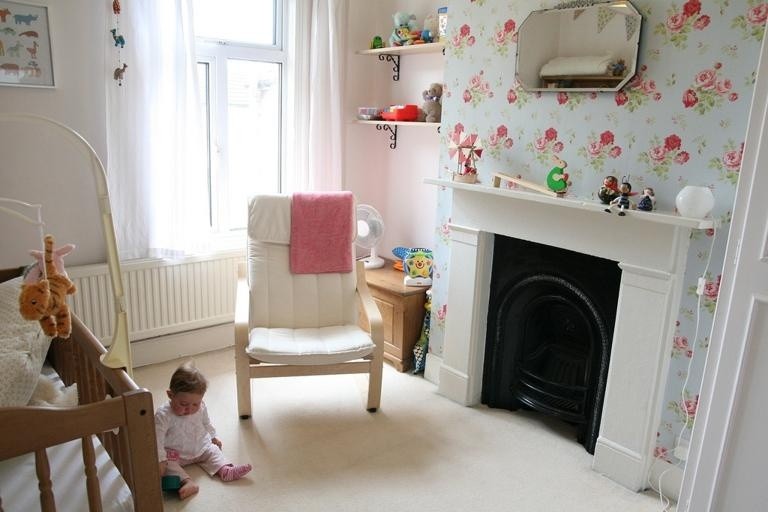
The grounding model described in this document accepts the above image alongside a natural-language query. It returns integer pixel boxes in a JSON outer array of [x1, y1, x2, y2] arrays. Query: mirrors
[[514, 2, 644, 92]]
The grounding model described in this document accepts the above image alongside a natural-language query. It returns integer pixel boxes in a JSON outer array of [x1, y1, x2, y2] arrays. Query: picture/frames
[[0, 1, 56, 89]]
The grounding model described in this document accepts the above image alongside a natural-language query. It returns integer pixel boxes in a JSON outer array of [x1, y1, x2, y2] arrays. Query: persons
[[154, 359, 253, 499]]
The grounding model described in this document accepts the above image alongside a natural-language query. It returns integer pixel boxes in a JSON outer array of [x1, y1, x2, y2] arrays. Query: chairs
[[235, 191, 384, 420]]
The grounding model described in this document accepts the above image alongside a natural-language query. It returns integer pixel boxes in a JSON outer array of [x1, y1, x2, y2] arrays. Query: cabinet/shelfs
[[354, 43, 446, 147], [357, 256, 431, 372]]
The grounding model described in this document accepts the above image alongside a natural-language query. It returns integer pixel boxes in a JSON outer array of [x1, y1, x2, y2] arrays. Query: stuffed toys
[[448, 129, 483, 185], [608, 57, 627, 75], [111, 29, 126, 50], [388, 11, 436, 46], [19, 234, 76, 339], [360, 82, 417, 122], [114, 63, 127, 87], [23, 244, 75, 286], [602, 173, 656, 216], [111, 1, 121, 14], [419, 81, 443, 123]]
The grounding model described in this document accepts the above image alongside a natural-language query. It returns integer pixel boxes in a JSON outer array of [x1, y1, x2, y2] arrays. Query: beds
[[0, 265, 165, 512]]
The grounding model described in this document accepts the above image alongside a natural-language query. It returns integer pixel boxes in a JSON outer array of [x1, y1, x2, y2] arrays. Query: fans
[[354, 204, 387, 270]]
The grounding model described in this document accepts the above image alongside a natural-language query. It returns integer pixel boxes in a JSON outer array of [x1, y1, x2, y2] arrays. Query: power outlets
[[674, 436, 691, 463]]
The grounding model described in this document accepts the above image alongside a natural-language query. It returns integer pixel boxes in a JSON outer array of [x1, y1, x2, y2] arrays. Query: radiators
[[66, 250, 237, 346]]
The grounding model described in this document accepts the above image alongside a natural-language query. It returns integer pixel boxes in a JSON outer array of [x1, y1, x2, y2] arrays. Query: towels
[[540, 54, 613, 75], [290, 192, 354, 273]]
